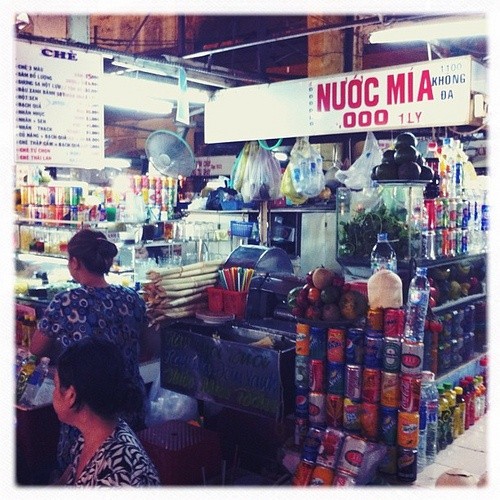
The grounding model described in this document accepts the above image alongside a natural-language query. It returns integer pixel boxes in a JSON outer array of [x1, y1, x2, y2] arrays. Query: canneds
[[124, 175, 175, 207], [422, 306, 476, 375], [22, 206, 78, 222], [290, 307, 422, 487], [419, 198, 490, 259], [20, 183, 83, 207]]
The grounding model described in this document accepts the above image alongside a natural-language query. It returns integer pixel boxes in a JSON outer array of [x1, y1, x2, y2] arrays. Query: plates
[[195, 310, 236, 324]]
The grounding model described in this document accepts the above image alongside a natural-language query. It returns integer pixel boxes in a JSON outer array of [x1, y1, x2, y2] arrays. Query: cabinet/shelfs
[[14, 208, 348, 311], [398, 252, 488, 393]]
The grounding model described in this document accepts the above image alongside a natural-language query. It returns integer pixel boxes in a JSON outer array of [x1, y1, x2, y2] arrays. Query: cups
[[224, 290, 249, 319]]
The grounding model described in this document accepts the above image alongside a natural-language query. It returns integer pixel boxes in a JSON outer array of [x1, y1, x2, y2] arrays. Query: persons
[[52, 335, 162, 486], [29, 230, 150, 474]]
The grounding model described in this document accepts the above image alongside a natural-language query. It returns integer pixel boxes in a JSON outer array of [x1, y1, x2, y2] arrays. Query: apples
[[290, 263, 366, 322]]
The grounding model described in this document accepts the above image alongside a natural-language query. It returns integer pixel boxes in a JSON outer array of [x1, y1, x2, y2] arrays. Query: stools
[[135, 419, 223, 486]]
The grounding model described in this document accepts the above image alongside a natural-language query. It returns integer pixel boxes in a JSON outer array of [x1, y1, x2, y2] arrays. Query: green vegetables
[[338, 204, 419, 263]]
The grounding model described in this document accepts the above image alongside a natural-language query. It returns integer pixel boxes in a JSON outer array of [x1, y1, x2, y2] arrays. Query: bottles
[[403, 267, 430, 345], [418, 356, 486, 470], [17, 348, 50, 408], [370, 233, 397, 274], [409, 137, 487, 263]]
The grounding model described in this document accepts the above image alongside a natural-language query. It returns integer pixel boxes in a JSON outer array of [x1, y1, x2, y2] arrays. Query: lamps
[[100, 57, 230, 115], [365, 17, 487, 44]]
